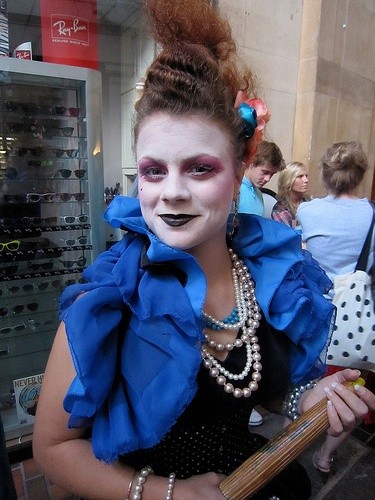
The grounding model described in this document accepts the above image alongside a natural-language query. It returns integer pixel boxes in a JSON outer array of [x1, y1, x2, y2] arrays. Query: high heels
[[313, 453, 333, 485]]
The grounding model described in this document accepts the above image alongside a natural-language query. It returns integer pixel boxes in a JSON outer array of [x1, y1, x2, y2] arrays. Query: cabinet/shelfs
[[0, 109, 86, 360]]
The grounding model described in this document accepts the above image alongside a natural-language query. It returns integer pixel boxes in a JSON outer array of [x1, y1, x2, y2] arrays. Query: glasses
[[0, 97, 95, 360]]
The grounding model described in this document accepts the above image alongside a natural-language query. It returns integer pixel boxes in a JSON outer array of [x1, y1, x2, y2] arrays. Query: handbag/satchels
[[325, 213, 375, 372]]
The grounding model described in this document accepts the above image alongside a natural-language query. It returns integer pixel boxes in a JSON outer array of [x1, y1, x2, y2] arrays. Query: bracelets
[[126, 467, 176, 500], [282, 380, 317, 422]]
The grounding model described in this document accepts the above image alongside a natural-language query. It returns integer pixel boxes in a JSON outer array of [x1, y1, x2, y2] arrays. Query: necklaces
[[199, 249, 261, 398]]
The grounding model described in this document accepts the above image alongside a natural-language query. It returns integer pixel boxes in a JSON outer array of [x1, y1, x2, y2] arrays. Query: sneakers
[[247, 409, 262, 426]]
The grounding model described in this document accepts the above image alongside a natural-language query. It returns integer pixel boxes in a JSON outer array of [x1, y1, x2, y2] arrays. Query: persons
[[33, 0, 375, 500], [229, 142, 375, 482]]
